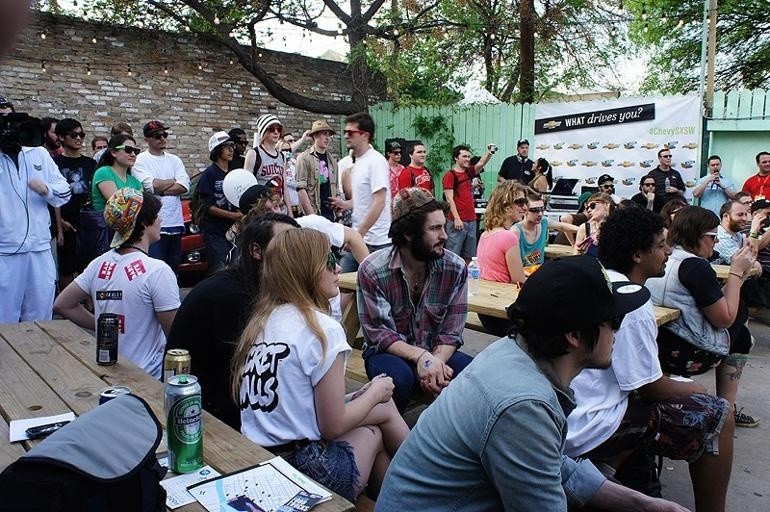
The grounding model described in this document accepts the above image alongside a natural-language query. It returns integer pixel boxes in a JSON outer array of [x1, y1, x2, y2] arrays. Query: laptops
[[540, 179, 578, 195]]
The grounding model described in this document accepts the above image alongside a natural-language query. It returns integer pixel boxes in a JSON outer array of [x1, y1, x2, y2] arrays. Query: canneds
[[98, 384, 131, 407], [163, 347, 192, 392], [164, 373, 204, 476]]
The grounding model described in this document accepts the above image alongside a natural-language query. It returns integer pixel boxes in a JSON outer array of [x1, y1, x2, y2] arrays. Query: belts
[[264, 439, 312, 452]]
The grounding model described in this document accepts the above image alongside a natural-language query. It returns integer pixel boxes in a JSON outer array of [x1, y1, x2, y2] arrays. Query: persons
[[160, 214, 412, 505], [354, 185, 475, 412], [191, 114, 554, 336], [560, 142, 768, 512], [372, 253, 687, 512], [0, 93, 189, 382]]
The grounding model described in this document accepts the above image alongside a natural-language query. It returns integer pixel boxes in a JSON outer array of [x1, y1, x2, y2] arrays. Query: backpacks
[[191, 168, 215, 224]]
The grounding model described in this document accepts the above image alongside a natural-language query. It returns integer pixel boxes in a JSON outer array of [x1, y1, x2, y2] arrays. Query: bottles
[[468, 256, 481, 297]]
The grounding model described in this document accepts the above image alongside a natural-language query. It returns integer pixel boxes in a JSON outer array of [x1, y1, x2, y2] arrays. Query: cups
[[490, 144, 497, 154]]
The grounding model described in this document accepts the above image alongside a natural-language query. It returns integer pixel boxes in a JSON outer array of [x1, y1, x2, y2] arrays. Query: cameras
[[761, 218, 770, 228], [490, 144, 498, 154], [714, 169, 719, 183]]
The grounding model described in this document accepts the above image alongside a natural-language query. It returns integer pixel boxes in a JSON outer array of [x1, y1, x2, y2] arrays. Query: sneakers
[[733, 404, 759, 427]]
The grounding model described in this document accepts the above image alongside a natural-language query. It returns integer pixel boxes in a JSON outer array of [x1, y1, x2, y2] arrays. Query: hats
[[751, 199, 770, 214], [104, 187, 144, 248], [144, 121, 169, 135], [257, 113, 281, 137], [310, 119, 334, 136], [578, 191, 592, 211], [598, 175, 614, 185], [516, 255, 651, 331], [208, 131, 235, 151], [392, 187, 434, 221]]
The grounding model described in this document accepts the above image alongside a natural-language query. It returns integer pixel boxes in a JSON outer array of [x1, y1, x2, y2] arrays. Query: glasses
[[586, 203, 596, 209], [392, 151, 401, 155], [601, 184, 614, 189], [529, 207, 544, 213], [644, 183, 655, 187], [344, 130, 368, 135], [515, 198, 528, 205], [663, 155, 672, 158], [70, 132, 84, 138], [268, 126, 282, 132], [699, 233, 718, 242], [327, 252, 337, 271], [155, 132, 168, 139], [115, 145, 140, 154]]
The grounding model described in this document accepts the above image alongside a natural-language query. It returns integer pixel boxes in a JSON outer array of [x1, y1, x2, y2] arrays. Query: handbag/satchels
[[0, 394, 167, 512], [77, 205, 111, 265]]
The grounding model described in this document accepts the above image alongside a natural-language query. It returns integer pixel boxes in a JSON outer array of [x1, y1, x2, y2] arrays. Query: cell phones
[[26, 421, 71, 439], [323, 201, 332, 209], [741, 233, 746, 247]]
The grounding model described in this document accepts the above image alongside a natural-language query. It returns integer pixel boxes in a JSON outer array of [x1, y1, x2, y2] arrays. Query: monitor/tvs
[[581, 186, 615, 194]]
